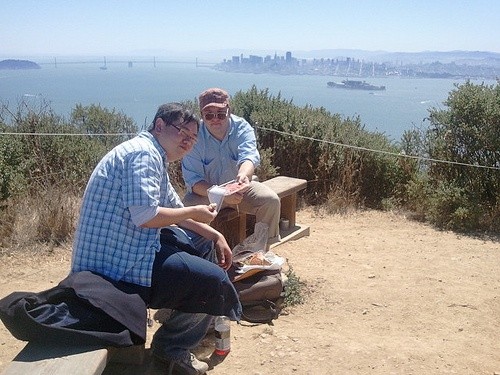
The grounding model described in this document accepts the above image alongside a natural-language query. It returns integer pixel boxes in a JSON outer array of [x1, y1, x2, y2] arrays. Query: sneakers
[[150, 349, 208, 375]]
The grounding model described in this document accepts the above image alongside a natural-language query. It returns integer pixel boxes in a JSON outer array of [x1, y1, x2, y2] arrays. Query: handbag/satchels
[[233, 273, 281, 322]]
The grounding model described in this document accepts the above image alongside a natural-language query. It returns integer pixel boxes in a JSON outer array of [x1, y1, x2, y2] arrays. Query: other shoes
[[264, 249, 284, 266]]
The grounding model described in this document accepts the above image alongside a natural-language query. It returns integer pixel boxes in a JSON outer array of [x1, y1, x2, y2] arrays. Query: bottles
[[215, 315, 231, 355]]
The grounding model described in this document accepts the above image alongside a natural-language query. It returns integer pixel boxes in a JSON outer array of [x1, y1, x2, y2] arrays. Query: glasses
[[204, 108, 229, 120], [161, 117, 199, 145]]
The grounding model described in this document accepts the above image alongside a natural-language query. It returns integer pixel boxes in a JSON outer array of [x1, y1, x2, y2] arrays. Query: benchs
[[209, 176, 310, 263], [0, 340, 145, 375]]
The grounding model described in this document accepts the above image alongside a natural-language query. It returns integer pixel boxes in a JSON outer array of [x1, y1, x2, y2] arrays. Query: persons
[[72, 103, 242, 375], [182, 88, 282, 252]]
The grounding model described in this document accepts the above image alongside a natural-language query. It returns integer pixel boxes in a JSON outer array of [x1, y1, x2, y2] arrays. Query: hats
[[199, 88, 228, 112]]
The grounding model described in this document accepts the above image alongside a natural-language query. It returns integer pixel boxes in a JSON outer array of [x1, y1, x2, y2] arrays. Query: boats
[[326, 77, 386, 91]]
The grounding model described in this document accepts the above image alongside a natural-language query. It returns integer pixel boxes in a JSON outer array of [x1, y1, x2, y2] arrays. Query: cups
[[281, 217, 290, 230]]
